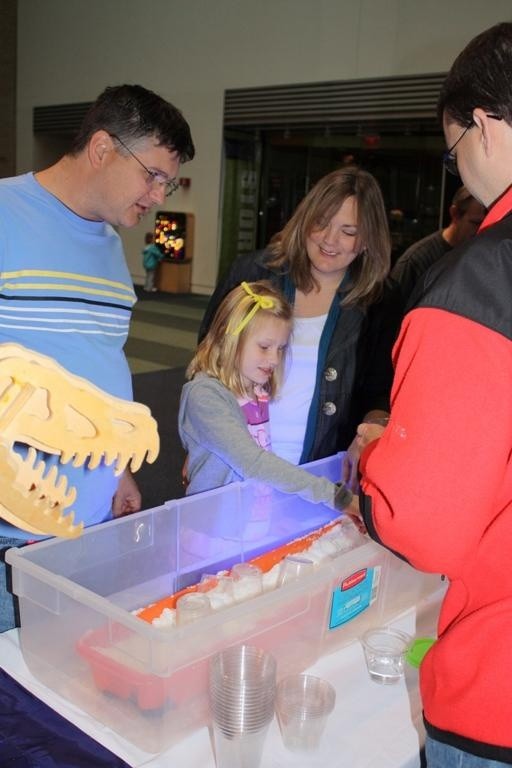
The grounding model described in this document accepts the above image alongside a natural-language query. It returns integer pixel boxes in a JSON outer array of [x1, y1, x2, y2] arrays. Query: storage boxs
[[5, 451, 450, 754]]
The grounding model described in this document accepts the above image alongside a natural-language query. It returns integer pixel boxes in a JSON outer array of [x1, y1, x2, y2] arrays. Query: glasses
[[442, 115, 503, 177], [108, 134, 179, 197]]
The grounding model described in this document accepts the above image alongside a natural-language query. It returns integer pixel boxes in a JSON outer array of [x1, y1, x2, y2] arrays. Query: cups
[[206, 641, 339, 767], [169, 555, 319, 629], [363, 626, 412, 683]]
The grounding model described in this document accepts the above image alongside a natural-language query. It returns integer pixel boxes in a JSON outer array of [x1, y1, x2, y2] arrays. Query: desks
[[0, 587, 448, 768]]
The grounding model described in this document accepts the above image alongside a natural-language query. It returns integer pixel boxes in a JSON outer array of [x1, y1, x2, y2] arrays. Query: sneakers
[[143, 287, 158, 293]]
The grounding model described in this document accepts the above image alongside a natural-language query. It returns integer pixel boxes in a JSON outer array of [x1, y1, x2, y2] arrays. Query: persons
[[1, 83, 197, 629], [140, 231, 168, 293], [181, 166, 394, 531], [175, 278, 362, 575], [387, 186, 488, 361], [343, 20, 512, 766]]
